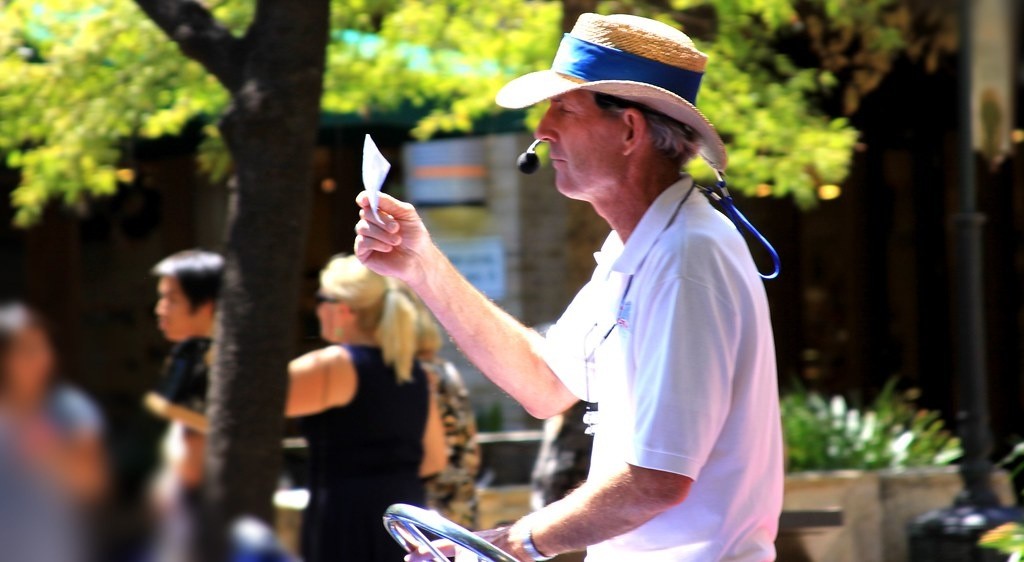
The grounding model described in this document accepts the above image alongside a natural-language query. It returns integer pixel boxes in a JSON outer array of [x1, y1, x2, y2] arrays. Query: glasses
[[314, 292, 342, 307]]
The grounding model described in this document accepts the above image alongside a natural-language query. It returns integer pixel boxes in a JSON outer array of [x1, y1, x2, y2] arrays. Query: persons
[[135, 251, 224, 562], [0, 300, 115, 562], [355, 13, 785, 562], [286, 254, 482, 562]]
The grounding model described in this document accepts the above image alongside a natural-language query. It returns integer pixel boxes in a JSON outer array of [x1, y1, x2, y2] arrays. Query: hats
[[495, 12, 726, 170]]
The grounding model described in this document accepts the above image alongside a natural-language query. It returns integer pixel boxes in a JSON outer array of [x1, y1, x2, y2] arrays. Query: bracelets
[[523, 512, 555, 561]]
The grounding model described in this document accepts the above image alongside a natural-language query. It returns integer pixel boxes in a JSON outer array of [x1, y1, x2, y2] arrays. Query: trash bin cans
[[904, 504, 1024, 562]]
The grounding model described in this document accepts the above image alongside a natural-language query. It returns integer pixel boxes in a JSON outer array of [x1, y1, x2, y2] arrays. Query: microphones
[[516, 138, 543, 175]]
